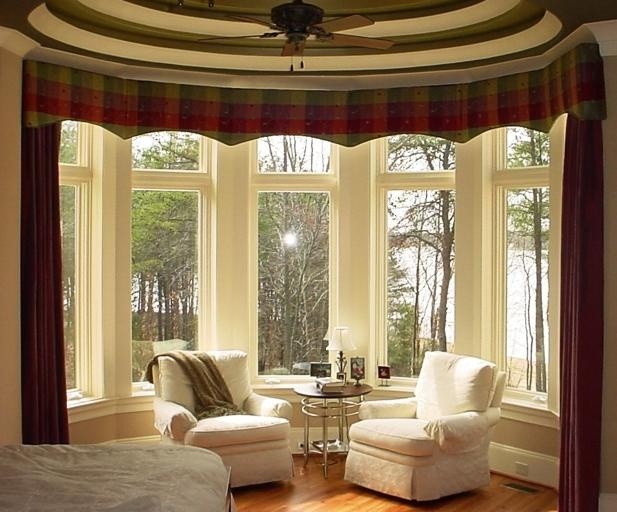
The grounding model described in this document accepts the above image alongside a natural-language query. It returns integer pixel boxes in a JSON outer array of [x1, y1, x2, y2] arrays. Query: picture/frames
[[378, 366, 390, 379], [350, 357, 365, 379]]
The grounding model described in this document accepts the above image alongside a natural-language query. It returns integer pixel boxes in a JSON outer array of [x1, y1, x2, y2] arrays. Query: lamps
[[326, 327, 357, 385], [286, 28, 307, 71]]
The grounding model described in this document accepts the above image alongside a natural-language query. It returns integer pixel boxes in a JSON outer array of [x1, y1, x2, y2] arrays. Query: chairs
[[343, 351, 506, 502], [151, 349, 294, 489]]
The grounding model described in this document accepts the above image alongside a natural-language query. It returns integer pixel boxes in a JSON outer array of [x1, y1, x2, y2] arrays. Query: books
[[319, 383, 344, 392], [316, 377, 345, 387]]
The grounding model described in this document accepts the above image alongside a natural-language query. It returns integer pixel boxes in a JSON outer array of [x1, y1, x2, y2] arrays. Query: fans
[[198, 0, 394, 56]]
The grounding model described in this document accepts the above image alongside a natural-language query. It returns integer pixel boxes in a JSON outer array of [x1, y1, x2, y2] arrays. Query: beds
[[0, 444, 236, 512]]
